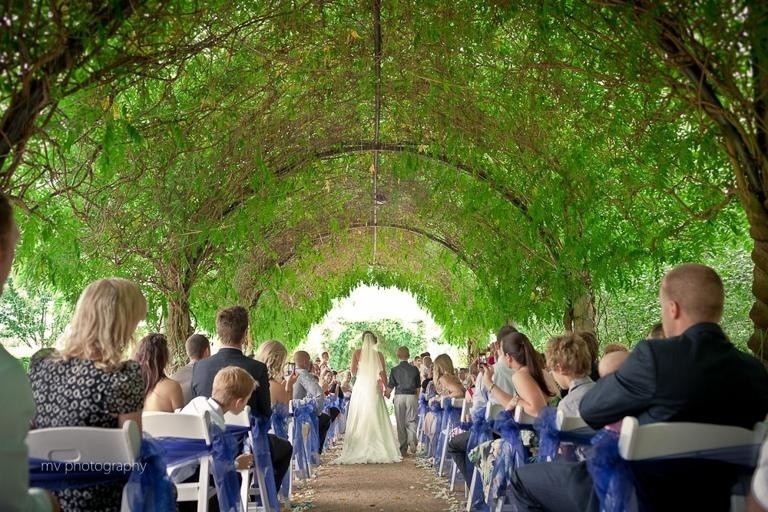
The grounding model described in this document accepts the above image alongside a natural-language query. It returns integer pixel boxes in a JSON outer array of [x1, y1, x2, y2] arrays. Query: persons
[[413, 265, 767, 511], [0, 192, 59, 512], [333, 332, 421, 463], [26, 278, 351, 512]]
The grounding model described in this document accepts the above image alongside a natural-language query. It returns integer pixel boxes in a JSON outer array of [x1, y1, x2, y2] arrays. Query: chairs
[[545, 409, 594, 465], [26, 419, 157, 512], [600, 415, 768, 512], [141, 409, 239, 512], [174, 405, 271, 512], [267, 392, 351, 502], [493, 406, 544, 512], [417, 400, 504, 511]]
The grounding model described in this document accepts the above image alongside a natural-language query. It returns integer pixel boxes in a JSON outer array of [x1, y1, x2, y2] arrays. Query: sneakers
[[409, 441, 416, 454], [401, 452, 408, 457], [463, 496, 485, 512]]
